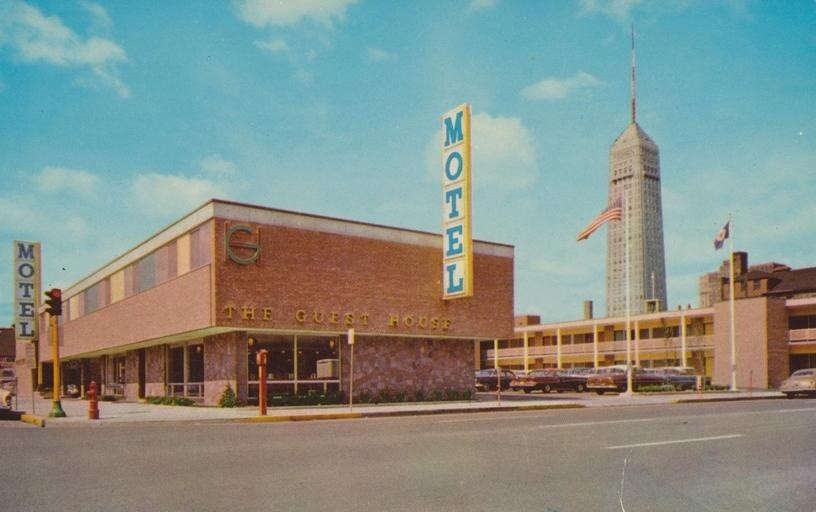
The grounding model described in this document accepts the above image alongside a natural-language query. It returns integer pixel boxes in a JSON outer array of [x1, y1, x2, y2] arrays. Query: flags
[[575, 194, 623, 243], [713, 221, 729, 251]]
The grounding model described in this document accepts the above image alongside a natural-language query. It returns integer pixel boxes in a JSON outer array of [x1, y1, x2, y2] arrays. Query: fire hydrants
[[85, 381, 100, 418]]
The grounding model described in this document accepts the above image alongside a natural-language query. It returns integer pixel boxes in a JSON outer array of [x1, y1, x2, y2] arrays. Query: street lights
[[256, 349, 268, 417]]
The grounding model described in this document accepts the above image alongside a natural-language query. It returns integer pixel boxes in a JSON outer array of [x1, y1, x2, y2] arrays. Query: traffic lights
[[50, 287, 61, 315], [44, 291, 52, 314]]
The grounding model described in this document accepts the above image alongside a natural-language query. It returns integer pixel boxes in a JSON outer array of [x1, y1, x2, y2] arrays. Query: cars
[[475, 364, 712, 392], [779, 368, 816, 396]]
